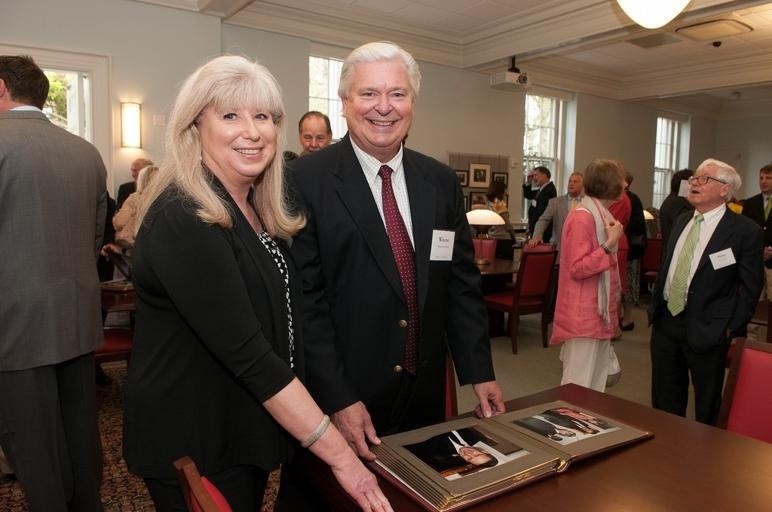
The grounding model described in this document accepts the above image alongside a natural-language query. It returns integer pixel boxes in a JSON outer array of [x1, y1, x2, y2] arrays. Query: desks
[[312, 382, 772, 512], [473, 257, 522, 339]]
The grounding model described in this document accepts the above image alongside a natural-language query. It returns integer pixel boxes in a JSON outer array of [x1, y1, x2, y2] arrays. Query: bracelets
[[300, 414, 331, 448]]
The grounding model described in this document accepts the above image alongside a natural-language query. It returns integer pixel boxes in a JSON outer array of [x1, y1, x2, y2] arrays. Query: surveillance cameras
[[490, 72, 532, 92], [712, 41, 721, 47]]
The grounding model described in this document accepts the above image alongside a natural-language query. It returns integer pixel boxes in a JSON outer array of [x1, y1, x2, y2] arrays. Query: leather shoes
[[618, 317, 635, 331]]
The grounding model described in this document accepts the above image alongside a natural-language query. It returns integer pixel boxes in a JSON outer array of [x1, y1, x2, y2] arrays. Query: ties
[[668, 214, 704, 316], [765, 197, 772, 219], [571, 197, 576, 210], [448, 430, 464, 446], [378, 165, 416, 375]]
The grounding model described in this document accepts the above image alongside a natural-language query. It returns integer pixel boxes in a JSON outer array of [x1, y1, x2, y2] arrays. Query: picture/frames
[[492, 171, 509, 189], [463, 195, 467, 214], [502, 191, 508, 210], [454, 169, 469, 188], [469, 190, 489, 212], [468, 162, 492, 189]]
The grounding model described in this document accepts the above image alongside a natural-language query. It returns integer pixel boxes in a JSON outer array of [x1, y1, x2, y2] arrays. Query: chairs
[[96, 327, 135, 388], [481, 251, 559, 354], [471, 237, 497, 262], [506, 240, 558, 331], [715, 336, 772, 444], [641, 239, 662, 304]]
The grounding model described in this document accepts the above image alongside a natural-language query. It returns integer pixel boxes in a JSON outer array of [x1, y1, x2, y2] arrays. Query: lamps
[[465, 209, 506, 266], [119, 101, 143, 149]]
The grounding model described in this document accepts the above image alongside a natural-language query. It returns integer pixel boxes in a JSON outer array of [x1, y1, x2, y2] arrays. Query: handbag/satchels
[[558, 342, 621, 389], [116, 216, 136, 249]]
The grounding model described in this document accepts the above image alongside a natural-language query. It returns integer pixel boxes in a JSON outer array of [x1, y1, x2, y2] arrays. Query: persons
[[484, 158, 647, 393], [407, 427, 498, 469], [0, 54, 108, 512], [121, 54, 394, 512], [512, 407, 611, 441], [647, 158, 772, 428], [96, 111, 332, 387], [275, 40, 505, 512]]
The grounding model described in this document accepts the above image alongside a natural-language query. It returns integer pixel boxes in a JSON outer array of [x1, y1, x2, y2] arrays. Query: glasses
[[689, 176, 726, 185]]
